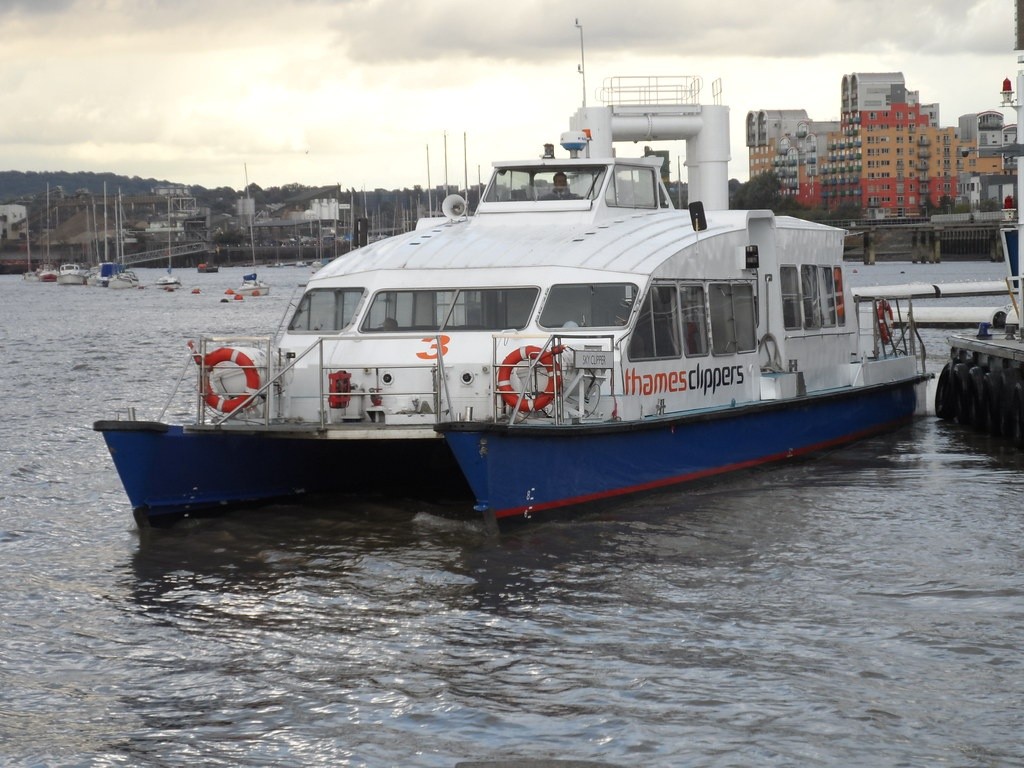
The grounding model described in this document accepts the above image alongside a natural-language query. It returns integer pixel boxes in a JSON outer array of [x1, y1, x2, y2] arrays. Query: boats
[[93, 19, 936, 536]]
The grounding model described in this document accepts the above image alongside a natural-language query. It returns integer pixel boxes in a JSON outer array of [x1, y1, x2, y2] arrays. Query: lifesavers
[[935, 362, 955, 419], [982, 372, 1007, 434], [198, 347, 261, 414], [498, 346, 562, 412], [878, 299, 894, 344], [952, 363, 970, 418], [1009, 382, 1024, 447], [967, 365, 986, 432]]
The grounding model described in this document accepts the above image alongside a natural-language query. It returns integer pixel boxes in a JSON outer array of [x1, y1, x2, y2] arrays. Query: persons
[[540, 170, 584, 200]]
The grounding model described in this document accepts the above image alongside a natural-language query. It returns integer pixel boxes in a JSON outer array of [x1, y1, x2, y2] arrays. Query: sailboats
[[270, 131, 481, 267], [24, 164, 139, 289], [157, 193, 181, 289], [238, 161, 270, 296]]
